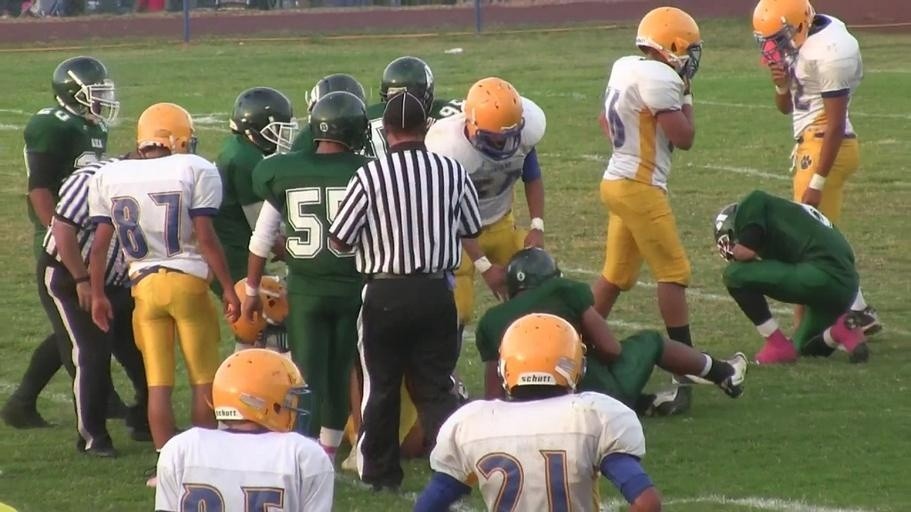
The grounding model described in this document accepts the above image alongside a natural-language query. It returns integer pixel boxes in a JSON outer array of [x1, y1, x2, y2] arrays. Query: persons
[[592, 5, 730, 384], [715, 189, 871, 365], [153, 349, 334, 512], [0, 57, 747, 488], [750, 1, 883, 350], [414, 312, 661, 511], [1, 1, 464, 18]]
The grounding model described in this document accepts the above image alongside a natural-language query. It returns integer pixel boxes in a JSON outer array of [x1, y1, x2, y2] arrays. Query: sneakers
[[755, 346, 796, 363], [87, 448, 118, 459], [719, 352, 749, 399], [849, 307, 881, 335], [3, 398, 50, 429], [646, 386, 691, 415], [835, 313, 868, 363], [672, 374, 713, 384], [126, 410, 151, 441], [105, 397, 130, 419]]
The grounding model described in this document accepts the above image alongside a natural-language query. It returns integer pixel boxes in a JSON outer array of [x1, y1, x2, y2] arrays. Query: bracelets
[[808, 173, 828, 192]]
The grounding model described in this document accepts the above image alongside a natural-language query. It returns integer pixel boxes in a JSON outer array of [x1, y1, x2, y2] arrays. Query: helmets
[[230, 87, 298, 154], [505, 247, 562, 298], [137, 103, 197, 154], [636, 7, 701, 78], [304, 73, 366, 111], [311, 91, 371, 152], [753, 0, 816, 69], [52, 57, 119, 127], [212, 348, 312, 437], [714, 203, 736, 263], [231, 275, 290, 352], [497, 312, 587, 394], [379, 57, 434, 113], [464, 77, 525, 159]]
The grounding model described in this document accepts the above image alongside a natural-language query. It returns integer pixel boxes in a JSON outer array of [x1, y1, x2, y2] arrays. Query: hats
[[382, 91, 427, 128]]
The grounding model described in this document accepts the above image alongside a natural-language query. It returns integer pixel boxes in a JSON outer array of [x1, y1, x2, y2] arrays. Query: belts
[[797, 132, 855, 143], [372, 272, 444, 279]]
